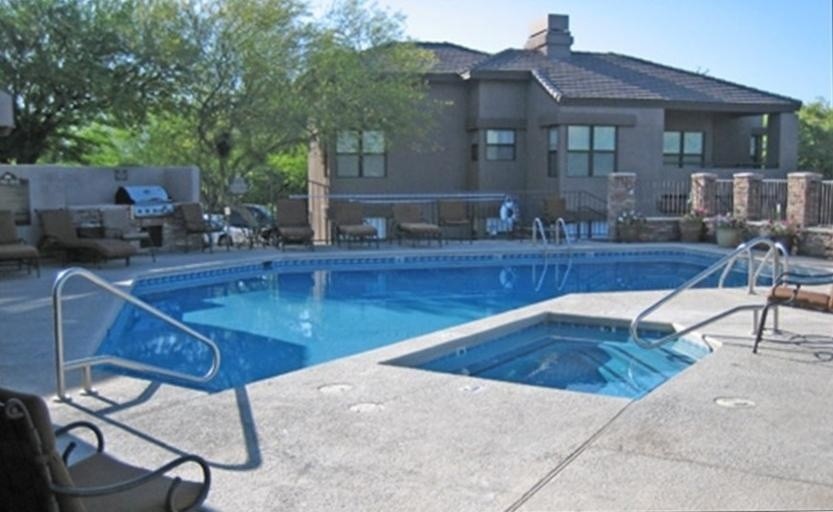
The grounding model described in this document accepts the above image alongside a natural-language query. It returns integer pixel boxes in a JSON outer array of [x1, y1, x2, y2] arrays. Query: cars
[[202, 203, 285, 247]]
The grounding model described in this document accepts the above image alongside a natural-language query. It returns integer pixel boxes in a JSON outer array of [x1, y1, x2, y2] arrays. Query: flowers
[[615, 208, 644, 225], [707, 210, 749, 234], [760, 215, 802, 244], [682, 207, 710, 223]]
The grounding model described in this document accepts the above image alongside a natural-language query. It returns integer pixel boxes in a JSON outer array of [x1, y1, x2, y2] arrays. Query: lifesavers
[[500, 202, 517, 220], [500, 269, 514, 288]]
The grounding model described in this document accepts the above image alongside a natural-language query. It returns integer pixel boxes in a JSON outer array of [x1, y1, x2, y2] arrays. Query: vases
[[771, 235, 793, 256], [714, 227, 741, 248], [616, 223, 640, 243], [678, 220, 706, 242]]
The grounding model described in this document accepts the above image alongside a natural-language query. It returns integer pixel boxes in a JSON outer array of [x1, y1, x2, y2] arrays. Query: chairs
[[178, 202, 230, 254], [0, 207, 41, 278], [752, 271, 833, 353], [33, 208, 135, 271], [517, 194, 607, 241], [273, 198, 315, 252], [0, 384, 211, 512], [438, 199, 473, 246], [96, 204, 158, 268], [327, 201, 380, 250], [387, 205, 444, 249]]
